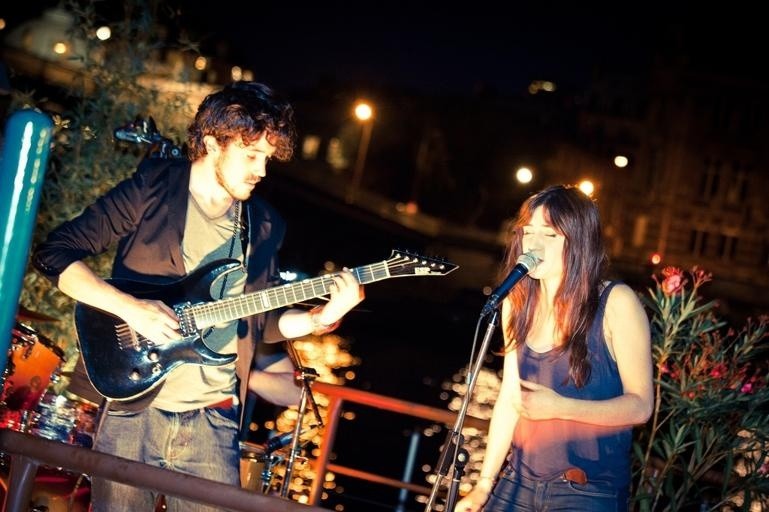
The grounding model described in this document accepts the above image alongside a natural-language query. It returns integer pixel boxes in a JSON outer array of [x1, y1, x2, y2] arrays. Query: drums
[[30, 391, 78, 442], [0, 402, 102, 512], [239, 444, 275, 495], [0, 320, 69, 411]]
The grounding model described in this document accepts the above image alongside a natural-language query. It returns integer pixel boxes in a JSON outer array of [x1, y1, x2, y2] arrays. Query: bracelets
[[476, 477, 497, 484], [310, 305, 342, 336]]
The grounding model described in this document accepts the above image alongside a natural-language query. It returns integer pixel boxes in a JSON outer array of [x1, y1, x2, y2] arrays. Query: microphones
[[267, 425, 317, 453], [480, 251, 539, 318]]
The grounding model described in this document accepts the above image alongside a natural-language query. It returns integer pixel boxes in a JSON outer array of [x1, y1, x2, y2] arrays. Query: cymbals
[[15, 304, 60, 322]]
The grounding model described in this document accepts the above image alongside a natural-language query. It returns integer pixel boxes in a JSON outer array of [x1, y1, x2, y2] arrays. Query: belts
[[563, 468, 585, 484], [207, 398, 232, 409]]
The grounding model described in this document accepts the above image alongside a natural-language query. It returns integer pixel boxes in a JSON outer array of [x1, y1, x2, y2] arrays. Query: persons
[[453, 186, 655, 512], [31, 81, 366, 512]]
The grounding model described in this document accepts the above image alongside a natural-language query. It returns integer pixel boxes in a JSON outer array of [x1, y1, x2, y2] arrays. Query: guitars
[[74, 250, 460, 400]]
